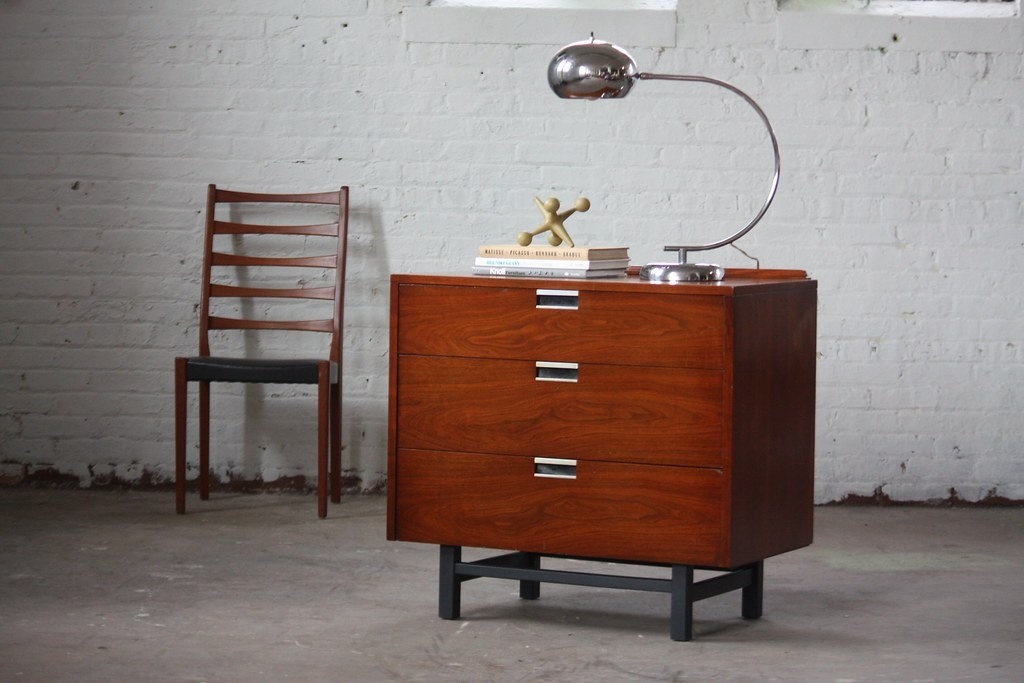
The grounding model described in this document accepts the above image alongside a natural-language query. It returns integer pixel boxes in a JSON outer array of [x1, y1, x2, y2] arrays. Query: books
[[472, 244, 631, 279]]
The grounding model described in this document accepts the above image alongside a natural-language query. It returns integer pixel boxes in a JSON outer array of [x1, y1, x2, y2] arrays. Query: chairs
[[173, 184, 349, 519]]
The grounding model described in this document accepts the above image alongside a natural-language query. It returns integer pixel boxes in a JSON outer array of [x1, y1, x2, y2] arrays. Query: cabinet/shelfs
[[385, 268, 819, 643]]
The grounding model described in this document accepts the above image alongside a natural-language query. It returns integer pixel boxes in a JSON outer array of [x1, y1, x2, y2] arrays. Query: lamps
[[548, 32, 780, 283]]
